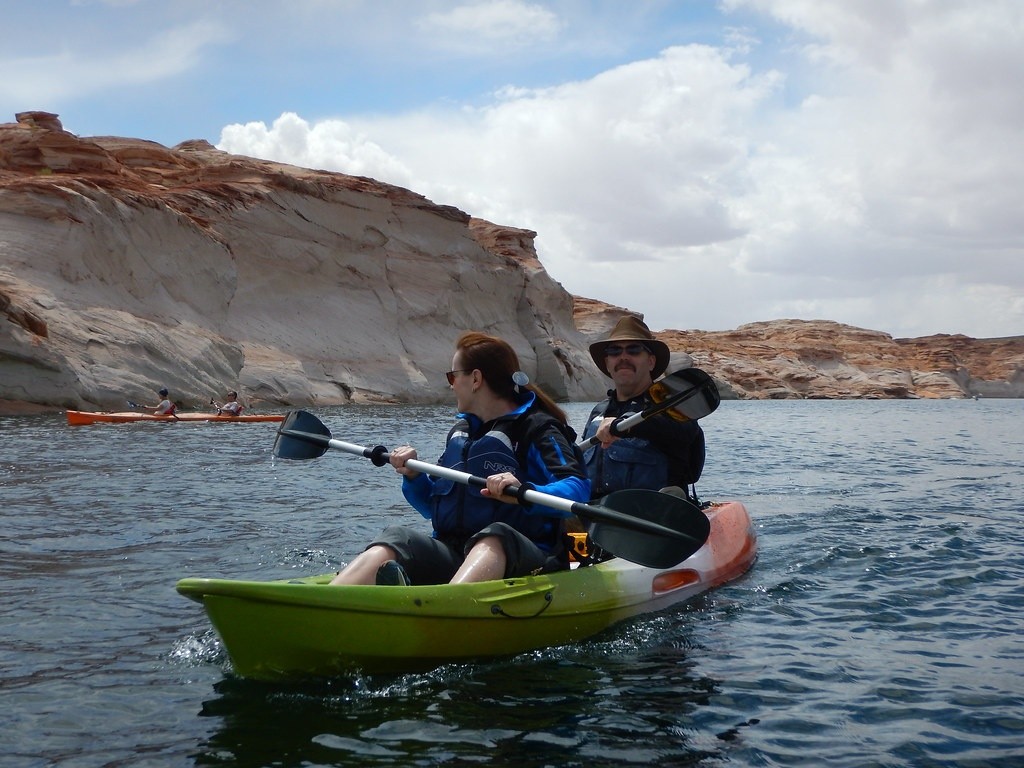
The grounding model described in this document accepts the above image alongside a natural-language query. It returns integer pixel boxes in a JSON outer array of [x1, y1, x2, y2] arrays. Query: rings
[[394, 449, 399, 452], [500, 476, 504, 479]]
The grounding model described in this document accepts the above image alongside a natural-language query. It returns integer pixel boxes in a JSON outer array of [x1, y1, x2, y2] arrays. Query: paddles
[[573, 367, 721, 452], [272, 409, 713, 571], [208, 396, 222, 415], [126, 400, 147, 410]]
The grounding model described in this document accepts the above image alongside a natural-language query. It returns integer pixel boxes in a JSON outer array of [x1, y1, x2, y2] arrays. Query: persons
[[327, 333, 592, 585], [576, 316, 705, 505], [145, 389, 173, 414], [217, 391, 238, 415]]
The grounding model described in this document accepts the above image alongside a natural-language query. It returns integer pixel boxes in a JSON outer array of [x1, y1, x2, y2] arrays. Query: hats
[[589, 315, 671, 380]]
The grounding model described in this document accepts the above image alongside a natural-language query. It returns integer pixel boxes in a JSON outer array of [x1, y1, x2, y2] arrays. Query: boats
[[62, 408, 289, 426], [175, 500, 759, 691]]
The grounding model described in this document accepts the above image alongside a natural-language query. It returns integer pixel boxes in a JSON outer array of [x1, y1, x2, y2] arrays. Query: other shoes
[[376, 560, 411, 586], [159, 389, 168, 397]]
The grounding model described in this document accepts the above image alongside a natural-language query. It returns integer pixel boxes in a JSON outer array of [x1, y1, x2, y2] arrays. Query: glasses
[[605, 345, 648, 357], [228, 395, 233, 397], [446, 369, 465, 385]]
